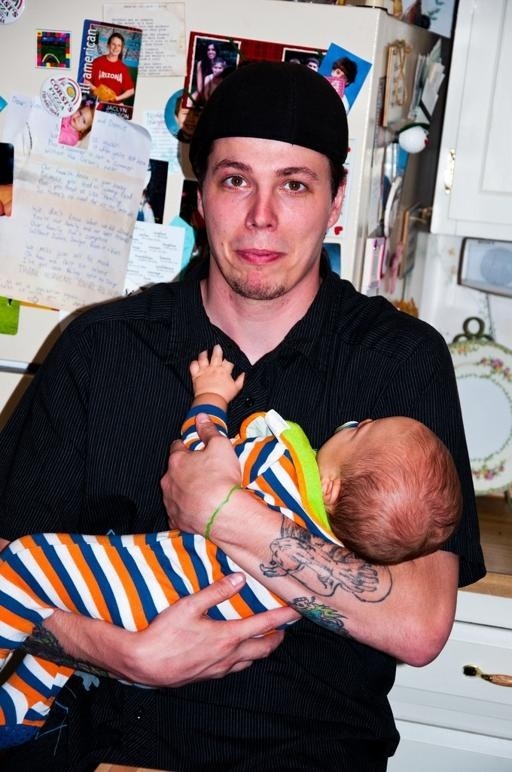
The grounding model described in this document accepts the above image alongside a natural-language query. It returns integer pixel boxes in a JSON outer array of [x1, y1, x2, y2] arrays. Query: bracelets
[[202, 483, 244, 548]]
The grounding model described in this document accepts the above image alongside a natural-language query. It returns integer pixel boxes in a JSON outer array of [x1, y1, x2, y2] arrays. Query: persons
[[324, 56, 358, 115], [0, 52, 487, 772], [57, 97, 95, 146], [306, 57, 320, 75], [0, 340, 473, 754], [172, 96, 203, 144], [84, 31, 137, 113], [204, 59, 227, 102], [135, 163, 157, 222], [190, 43, 224, 102]]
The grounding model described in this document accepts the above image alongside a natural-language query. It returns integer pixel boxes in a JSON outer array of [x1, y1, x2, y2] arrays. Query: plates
[[446, 341, 512, 501]]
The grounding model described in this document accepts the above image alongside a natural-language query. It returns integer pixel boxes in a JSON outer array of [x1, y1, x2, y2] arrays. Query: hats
[[190, 61, 350, 168]]
